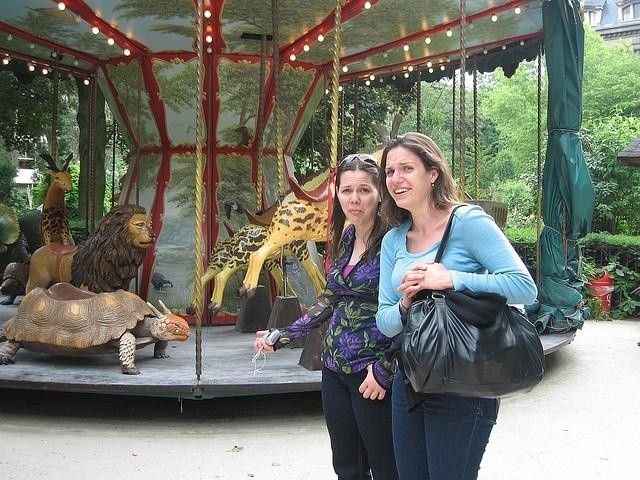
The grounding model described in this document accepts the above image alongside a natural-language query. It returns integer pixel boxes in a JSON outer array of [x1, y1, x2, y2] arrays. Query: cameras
[[258, 329, 280, 346]]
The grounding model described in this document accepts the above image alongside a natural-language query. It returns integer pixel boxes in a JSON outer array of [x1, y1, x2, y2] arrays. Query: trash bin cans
[[587, 269, 614, 316]]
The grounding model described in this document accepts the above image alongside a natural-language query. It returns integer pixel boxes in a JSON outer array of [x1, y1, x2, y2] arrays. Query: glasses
[[336, 154, 379, 168]]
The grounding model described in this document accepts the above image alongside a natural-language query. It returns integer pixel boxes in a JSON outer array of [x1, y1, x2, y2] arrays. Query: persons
[[374, 130, 539, 480], [253, 154, 403, 480]]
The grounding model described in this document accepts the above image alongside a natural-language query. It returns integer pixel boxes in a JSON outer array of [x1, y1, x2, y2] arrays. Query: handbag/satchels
[[397, 288, 544, 399]]
